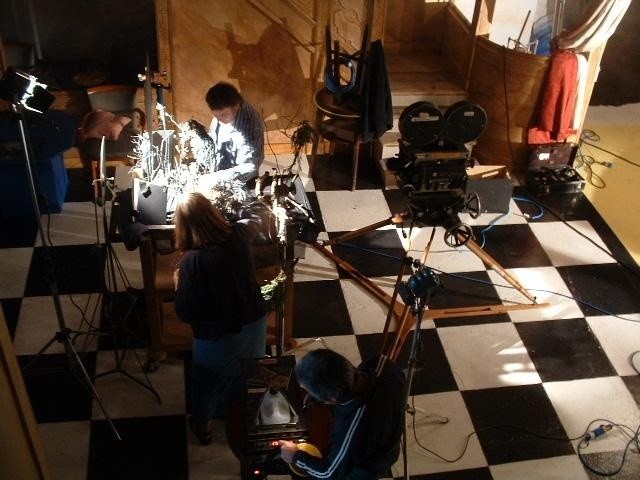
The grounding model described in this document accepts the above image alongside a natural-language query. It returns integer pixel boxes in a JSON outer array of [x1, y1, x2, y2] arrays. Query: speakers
[[460, 164, 512, 213]]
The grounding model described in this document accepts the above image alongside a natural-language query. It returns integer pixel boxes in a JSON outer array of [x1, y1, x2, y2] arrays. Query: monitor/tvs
[[132, 178, 167, 226]]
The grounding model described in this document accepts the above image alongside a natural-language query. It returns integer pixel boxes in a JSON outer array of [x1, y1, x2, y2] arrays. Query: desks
[[129, 156, 308, 361], [309, 86, 373, 192]]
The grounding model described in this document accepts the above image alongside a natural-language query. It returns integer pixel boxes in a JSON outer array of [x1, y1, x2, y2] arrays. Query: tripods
[[91, 206, 163, 407], [405, 300, 449, 480], [18, 114, 124, 448]]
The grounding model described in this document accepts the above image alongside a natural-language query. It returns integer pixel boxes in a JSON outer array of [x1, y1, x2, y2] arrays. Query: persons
[[173, 191, 269, 446], [198, 81, 264, 192], [278, 348, 407, 480]]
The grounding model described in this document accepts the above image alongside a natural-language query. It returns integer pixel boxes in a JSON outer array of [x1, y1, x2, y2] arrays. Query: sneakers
[[189, 416, 213, 446]]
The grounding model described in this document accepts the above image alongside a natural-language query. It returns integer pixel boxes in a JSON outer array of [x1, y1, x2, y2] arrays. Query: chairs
[[78, 85, 158, 197], [90, 159, 133, 242]]
